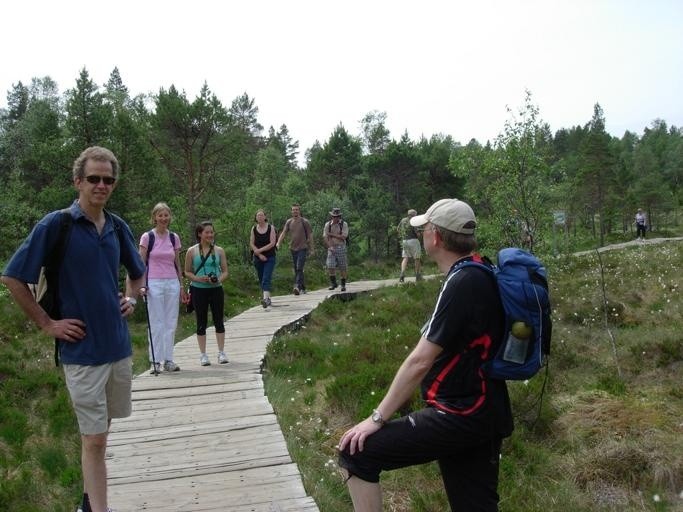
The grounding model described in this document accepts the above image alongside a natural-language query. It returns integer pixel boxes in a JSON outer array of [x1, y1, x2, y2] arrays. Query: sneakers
[[260, 298, 272, 309], [217, 351, 230, 364], [398, 271, 426, 283], [200, 354, 211, 366], [149, 363, 161, 375], [164, 361, 181, 372], [293, 286, 309, 296]]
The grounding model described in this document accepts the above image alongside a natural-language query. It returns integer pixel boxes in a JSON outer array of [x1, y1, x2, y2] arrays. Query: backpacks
[[28, 207, 123, 368], [446, 247, 553, 384]]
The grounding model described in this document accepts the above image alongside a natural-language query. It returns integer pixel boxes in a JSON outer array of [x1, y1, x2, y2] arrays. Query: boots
[[328, 274, 347, 291]]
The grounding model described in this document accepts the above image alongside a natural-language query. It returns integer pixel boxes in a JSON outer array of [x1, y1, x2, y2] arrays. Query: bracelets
[[180, 285, 185, 288]]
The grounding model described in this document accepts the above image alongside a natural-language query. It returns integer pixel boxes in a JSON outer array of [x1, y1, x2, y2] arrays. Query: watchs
[[124, 295, 137, 307], [372, 409, 386, 426]]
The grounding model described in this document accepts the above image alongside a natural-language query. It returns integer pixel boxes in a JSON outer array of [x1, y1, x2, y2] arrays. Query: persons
[[276, 205, 313, 295], [337, 199, 514, 511], [184, 221, 229, 365], [322, 207, 349, 292], [397, 209, 426, 283], [504, 208, 536, 257], [249, 209, 277, 306], [631, 207, 647, 243], [1, 147, 145, 511], [139, 202, 188, 375]]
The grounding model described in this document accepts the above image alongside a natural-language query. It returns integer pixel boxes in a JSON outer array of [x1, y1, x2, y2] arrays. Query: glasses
[[82, 175, 117, 186]]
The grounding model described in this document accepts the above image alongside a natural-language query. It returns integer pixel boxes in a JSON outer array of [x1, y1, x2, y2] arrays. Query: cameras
[[208, 272, 217, 283]]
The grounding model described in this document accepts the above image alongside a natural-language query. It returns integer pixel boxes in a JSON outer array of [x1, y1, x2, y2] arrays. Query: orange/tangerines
[[512, 321, 533, 339]]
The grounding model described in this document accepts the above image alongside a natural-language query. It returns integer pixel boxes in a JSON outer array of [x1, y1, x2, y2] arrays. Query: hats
[[409, 197, 477, 235], [328, 207, 344, 216]]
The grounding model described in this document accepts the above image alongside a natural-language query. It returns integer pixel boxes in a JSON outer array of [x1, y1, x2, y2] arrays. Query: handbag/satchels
[[185, 287, 195, 315]]
